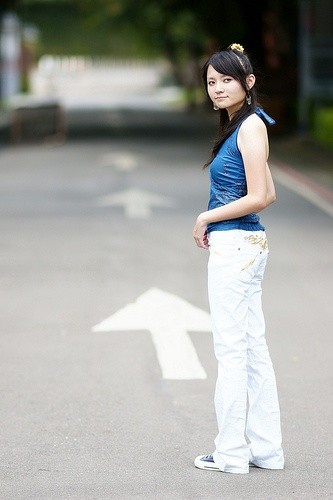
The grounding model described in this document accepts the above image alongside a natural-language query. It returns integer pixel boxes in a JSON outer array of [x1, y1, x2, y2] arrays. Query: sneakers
[[249, 461, 255, 466], [194, 455, 220, 471]]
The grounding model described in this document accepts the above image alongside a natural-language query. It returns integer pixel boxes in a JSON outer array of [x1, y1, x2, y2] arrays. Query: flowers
[[231, 43, 244, 53]]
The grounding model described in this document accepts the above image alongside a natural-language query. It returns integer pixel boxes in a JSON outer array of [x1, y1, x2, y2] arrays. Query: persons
[[193, 42, 284, 474]]
